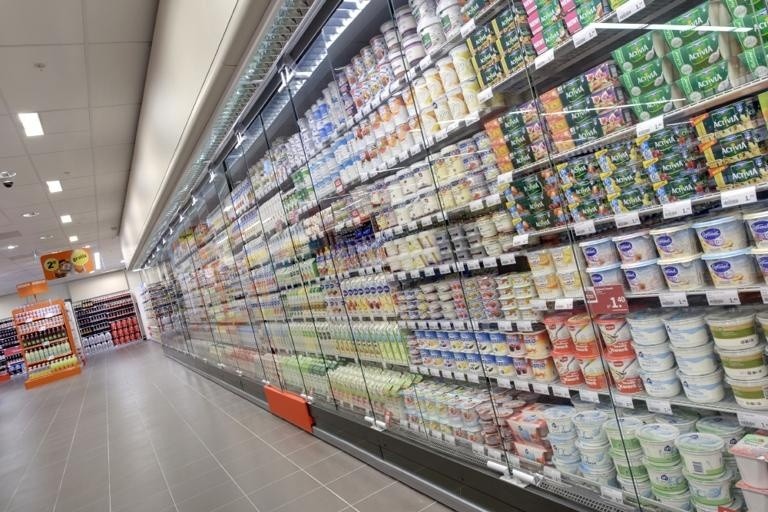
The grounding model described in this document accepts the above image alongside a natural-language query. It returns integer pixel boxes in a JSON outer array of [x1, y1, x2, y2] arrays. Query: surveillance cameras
[[2, 181, 13, 189]]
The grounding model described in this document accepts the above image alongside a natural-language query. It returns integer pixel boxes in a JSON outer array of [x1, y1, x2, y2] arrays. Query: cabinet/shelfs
[[71, 292, 147, 358], [11, 278, 81, 390], [129, 0, 767, 512], [0, 317, 27, 382]]
